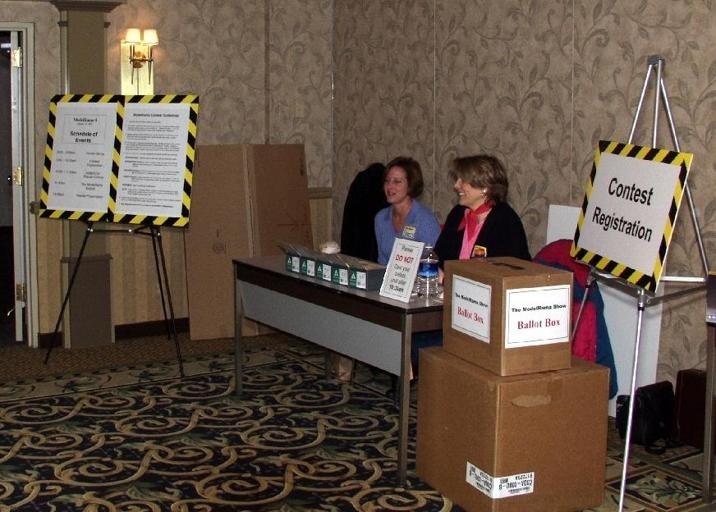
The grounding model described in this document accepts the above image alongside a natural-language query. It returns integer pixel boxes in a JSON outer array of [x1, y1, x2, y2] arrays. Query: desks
[[232, 251, 445, 487]]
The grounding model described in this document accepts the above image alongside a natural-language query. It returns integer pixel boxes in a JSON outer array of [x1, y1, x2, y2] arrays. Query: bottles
[[415, 242, 441, 299]]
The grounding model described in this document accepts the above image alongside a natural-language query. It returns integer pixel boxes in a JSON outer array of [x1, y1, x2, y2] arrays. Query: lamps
[[120, 28, 160, 85]]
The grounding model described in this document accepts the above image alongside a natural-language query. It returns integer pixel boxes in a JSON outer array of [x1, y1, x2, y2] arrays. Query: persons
[[412, 154, 532, 349], [374, 156, 442, 395]]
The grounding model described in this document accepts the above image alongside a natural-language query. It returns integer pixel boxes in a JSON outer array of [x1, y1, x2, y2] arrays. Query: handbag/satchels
[[618, 380, 675, 444]]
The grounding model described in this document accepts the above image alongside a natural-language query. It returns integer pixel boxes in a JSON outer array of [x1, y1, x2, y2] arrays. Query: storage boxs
[[413, 346, 611, 511], [443, 256, 573, 377]]
